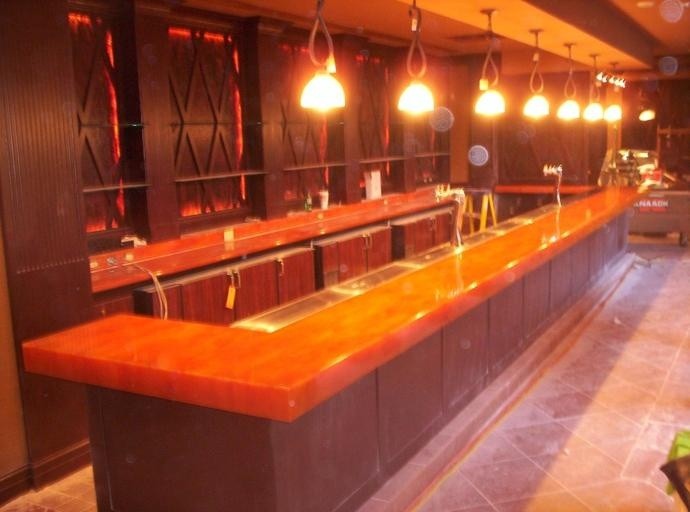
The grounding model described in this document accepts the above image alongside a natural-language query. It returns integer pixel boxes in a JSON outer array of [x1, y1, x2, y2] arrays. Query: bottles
[[305, 192, 312, 211], [450, 223, 462, 247], [319, 184, 328, 208], [435, 181, 451, 198]]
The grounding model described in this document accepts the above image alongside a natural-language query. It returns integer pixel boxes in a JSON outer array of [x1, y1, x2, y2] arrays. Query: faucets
[[542, 163, 563, 206], [433, 183, 468, 247]]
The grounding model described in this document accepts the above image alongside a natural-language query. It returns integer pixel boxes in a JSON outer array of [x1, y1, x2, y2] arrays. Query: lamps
[[584, 53, 603, 123], [525, 28, 550, 119], [558, 43, 580, 122], [605, 62, 623, 123], [474, 9, 507, 117], [394, 1, 434, 117], [299, 0, 347, 116]]
